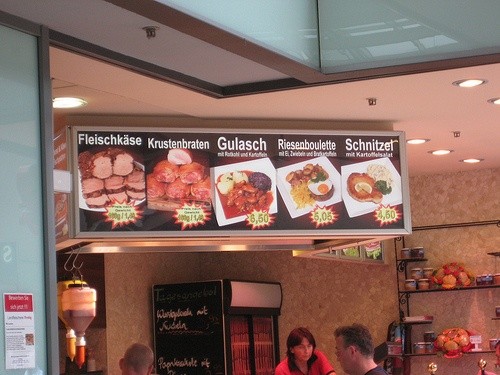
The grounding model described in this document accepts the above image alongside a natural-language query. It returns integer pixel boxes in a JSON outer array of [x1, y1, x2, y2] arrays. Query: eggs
[[307, 177, 333, 196]]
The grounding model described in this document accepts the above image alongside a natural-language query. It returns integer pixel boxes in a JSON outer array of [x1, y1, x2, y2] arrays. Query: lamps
[[61, 286, 97, 368], [57, 279, 90, 361]]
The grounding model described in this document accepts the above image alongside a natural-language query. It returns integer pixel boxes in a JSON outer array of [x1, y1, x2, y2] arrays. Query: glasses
[[334, 349, 351, 358]]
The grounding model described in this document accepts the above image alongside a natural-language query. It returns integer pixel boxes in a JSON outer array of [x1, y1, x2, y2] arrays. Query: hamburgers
[[431, 261, 472, 290], [435, 327, 477, 358]]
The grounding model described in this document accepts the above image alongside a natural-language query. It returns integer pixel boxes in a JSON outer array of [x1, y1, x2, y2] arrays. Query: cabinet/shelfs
[[393, 220, 500, 375], [150, 278, 283, 375]]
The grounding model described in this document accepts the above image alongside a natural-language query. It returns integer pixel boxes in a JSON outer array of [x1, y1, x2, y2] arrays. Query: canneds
[[405, 268, 434, 290], [412, 331, 435, 355], [489, 338, 498, 350], [476, 274, 500, 286], [495, 307, 500, 317], [401, 246, 425, 258]]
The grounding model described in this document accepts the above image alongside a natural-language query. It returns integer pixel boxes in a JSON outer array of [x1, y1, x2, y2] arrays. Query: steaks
[[347, 173, 383, 203]]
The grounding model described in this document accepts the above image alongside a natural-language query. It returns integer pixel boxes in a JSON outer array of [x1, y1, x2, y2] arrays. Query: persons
[[273, 327, 336, 375], [333, 322, 390, 375], [119, 343, 154, 375]]
